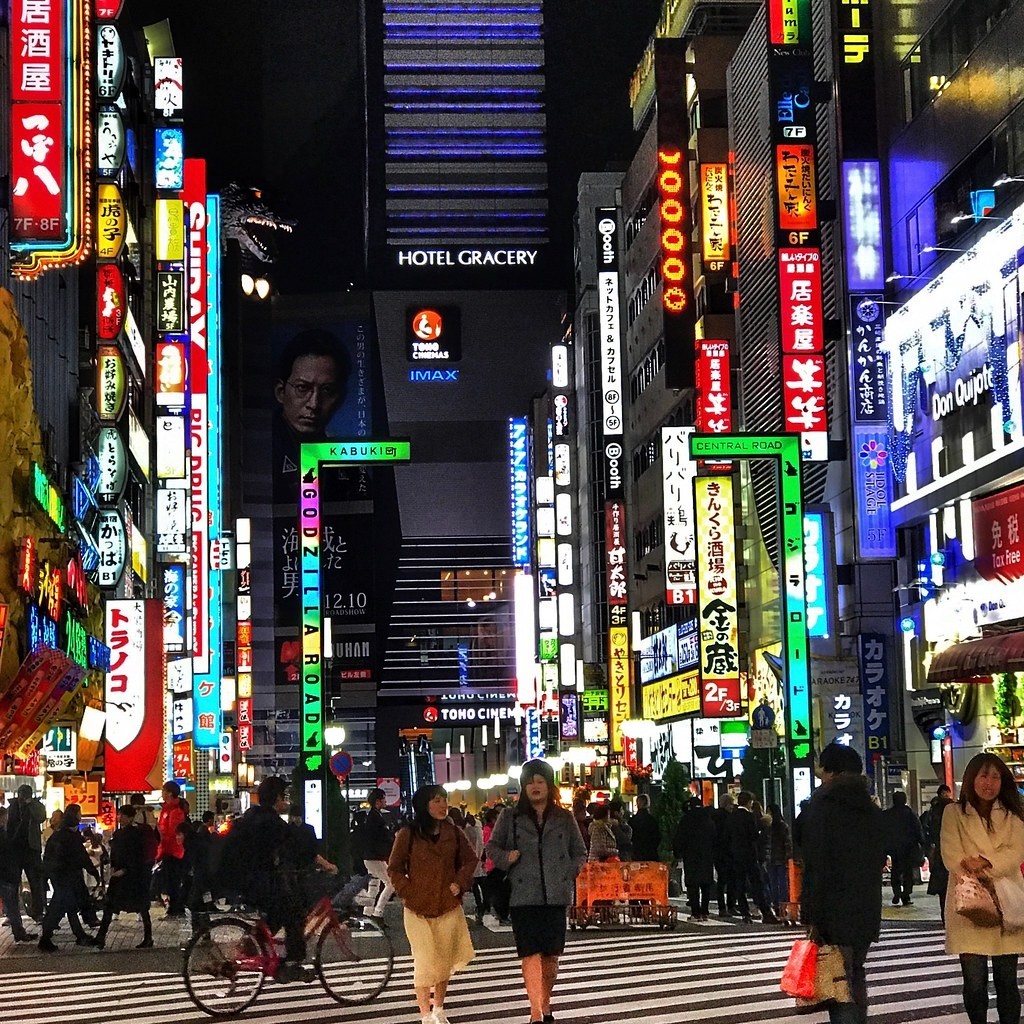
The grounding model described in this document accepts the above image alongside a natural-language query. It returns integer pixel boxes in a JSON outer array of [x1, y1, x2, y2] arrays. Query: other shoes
[[499, 918, 513, 926], [530, 1010, 554, 1024], [36, 919, 43, 925], [370, 914, 392, 928], [422, 1013, 438, 1024], [2, 918, 22, 926], [239, 933, 268, 957], [571, 905, 652, 925], [88, 919, 102, 929], [158, 914, 178, 921], [135, 939, 154, 948], [892, 894, 901, 904], [89, 934, 106, 948], [14, 931, 39, 942], [685, 903, 790, 928], [433, 1006, 450, 1024], [903, 902, 914, 905], [37, 936, 58, 952], [75, 933, 93, 947], [273, 963, 315, 984], [180, 932, 212, 952]]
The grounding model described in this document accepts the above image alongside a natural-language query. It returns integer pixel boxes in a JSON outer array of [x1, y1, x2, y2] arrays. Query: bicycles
[[180, 869, 395, 1018]]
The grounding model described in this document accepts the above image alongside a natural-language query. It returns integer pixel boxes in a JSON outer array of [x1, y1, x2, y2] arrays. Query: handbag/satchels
[[781, 938, 818, 998], [99, 844, 110, 864], [954, 869, 1002, 927], [983, 868, 1024, 931], [795, 944, 853, 1007], [481, 867, 512, 904]]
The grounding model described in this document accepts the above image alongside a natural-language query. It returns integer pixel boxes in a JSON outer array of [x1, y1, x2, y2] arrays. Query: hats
[[258, 776, 290, 800], [520, 759, 554, 787], [892, 791, 907, 805]]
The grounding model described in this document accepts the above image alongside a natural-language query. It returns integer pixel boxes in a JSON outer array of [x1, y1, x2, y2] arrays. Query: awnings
[[926, 630, 1024, 684]]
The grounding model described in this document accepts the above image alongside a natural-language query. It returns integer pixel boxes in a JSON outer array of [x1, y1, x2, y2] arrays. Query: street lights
[[324, 724, 346, 758], [443, 610, 655, 814]]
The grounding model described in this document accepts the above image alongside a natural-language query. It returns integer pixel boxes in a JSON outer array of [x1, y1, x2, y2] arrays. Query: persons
[[0, 775, 344, 984], [941, 753, 1024, 1024], [800, 744, 888, 1024], [386, 784, 480, 1024], [243, 332, 357, 500], [487, 759, 588, 1024], [332, 783, 959, 930]]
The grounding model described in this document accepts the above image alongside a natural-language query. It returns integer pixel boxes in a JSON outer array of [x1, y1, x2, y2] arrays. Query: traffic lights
[[763, 778, 783, 816]]
